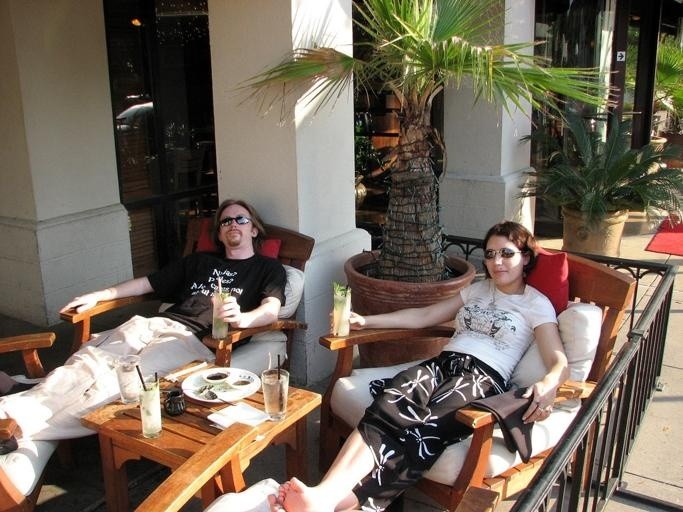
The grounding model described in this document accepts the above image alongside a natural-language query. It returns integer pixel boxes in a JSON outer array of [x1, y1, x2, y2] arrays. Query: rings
[[538, 406, 544, 412]]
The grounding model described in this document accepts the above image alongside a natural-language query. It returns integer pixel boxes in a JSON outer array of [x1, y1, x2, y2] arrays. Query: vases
[[342, 250, 478, 364]]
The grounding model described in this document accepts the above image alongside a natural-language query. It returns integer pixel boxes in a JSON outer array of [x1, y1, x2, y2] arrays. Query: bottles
[[165, 391, 186, 416]]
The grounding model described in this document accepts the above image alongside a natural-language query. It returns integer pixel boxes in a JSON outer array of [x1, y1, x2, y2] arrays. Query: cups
[[113, 355, 163, 440], [333, 287, 351, 338], [262, 369, 290, 421], [212, 292, 231, 340]]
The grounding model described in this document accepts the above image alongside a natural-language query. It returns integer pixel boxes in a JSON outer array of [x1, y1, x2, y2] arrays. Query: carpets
[[644, 214, 683, 256]]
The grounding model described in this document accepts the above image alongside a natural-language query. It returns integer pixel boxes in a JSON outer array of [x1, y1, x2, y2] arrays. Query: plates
[[183, 366, 261, 404], [207, 402, 268, 433]]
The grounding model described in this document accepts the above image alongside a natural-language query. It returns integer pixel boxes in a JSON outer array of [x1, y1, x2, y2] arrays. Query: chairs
[[57, 216, 317, 459], [0, 325, 60, 458], [313, 239, 636, 509], [125, 417, 258, 512]]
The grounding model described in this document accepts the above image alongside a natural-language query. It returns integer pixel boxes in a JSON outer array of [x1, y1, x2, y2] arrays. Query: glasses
[[219, 216, 249, 227], [483, 247, 523, 260]]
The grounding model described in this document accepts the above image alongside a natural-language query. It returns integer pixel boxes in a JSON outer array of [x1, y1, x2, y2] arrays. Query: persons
[[268, 220, 571, 512], [0, 198, 287, 499]]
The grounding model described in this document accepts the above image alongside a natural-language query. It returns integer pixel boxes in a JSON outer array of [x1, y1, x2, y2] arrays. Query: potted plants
[[512, 97, 683, 266], [223, 0, 621, 369]]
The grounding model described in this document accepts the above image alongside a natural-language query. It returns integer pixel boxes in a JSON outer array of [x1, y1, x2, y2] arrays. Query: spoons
[[203, 389, 238, 407]]
